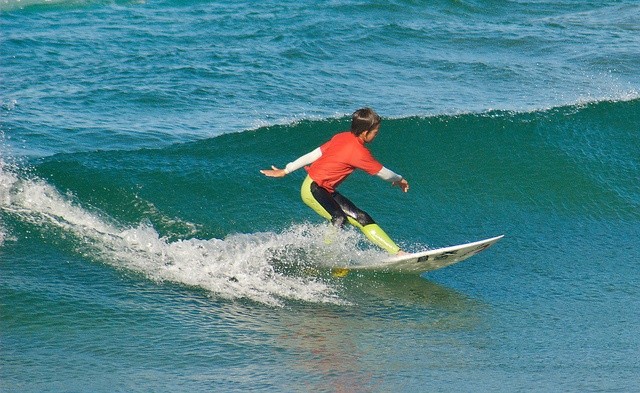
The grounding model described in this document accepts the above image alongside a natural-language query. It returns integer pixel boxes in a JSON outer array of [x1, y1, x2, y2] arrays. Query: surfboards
[[335, 234, 505, 274]]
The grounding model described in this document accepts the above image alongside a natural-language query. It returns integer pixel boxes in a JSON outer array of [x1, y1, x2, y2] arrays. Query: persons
[[260, 108, 411, 257]]
